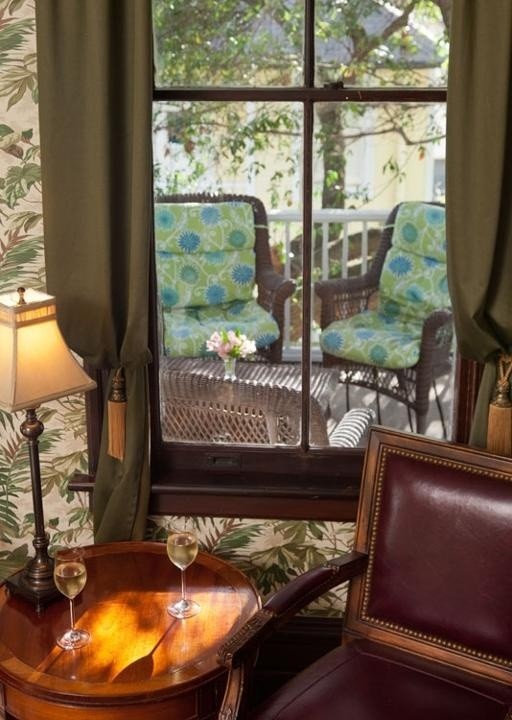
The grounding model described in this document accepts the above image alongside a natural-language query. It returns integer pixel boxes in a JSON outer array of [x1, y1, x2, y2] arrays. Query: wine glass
[[164, 531, 200, 619], [50, 549, 92, 654]]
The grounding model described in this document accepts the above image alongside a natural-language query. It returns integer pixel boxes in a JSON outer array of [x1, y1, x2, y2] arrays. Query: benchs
[[159, 368, 377, 448]]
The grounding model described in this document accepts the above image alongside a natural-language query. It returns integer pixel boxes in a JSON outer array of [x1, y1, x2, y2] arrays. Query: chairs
[[154, 192, 297, 362], [314, 200, 455, 436], [216, 424, 512, 720]]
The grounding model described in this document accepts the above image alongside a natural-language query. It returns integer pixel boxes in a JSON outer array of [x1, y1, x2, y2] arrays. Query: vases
[[223, 358, 237, 383]]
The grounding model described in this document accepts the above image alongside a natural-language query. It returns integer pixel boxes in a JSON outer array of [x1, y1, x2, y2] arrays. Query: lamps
[[0, 287, 97, 615]]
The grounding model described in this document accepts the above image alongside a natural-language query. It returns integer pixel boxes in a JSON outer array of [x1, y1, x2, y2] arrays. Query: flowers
[[205, 328, 257, 370]]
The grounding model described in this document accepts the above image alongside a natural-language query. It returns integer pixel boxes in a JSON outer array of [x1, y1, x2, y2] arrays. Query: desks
[[159, 356, 340, 418]]
